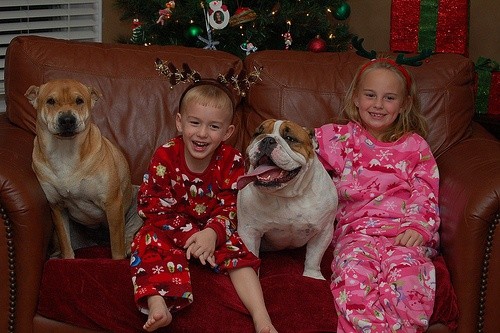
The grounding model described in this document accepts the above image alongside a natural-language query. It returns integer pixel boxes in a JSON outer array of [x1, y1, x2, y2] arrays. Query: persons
[[130, 83, 282, 333], [311, 56, 441, 333]]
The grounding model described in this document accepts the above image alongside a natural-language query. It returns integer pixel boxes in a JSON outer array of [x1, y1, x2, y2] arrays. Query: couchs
[[0, 36, 500, 333]]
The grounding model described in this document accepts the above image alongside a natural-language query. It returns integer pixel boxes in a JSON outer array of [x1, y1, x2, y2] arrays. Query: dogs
[[25, 80, 133, 260], [236, 119, 339, 281]]
